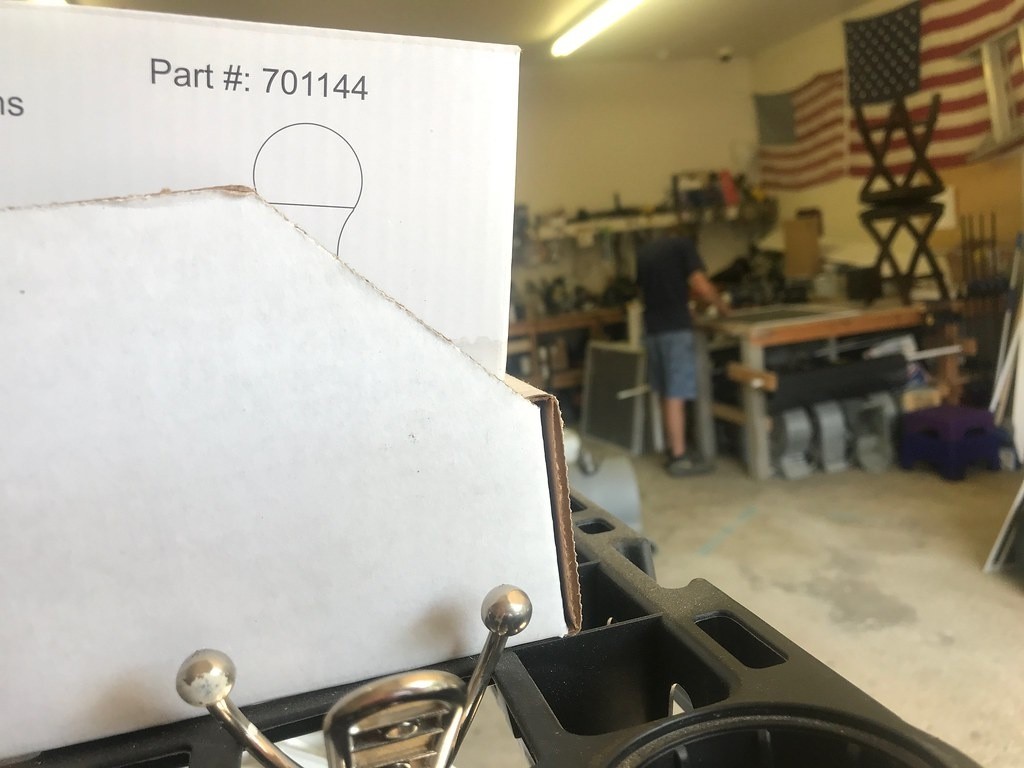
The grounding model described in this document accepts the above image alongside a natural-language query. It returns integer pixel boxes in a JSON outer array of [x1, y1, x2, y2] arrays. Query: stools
[[858, 203, 950, 306], [849, 90, 945, 203]]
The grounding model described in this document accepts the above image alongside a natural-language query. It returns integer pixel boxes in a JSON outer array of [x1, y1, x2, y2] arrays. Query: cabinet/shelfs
[[506, 306, 624, 390]]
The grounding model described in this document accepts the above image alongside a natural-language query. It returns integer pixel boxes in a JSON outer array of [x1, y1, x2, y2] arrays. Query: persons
[[635, 222, 731, 478]]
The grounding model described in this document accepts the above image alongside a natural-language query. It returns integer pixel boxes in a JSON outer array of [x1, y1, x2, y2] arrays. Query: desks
[[691, 287, 1010, 479]]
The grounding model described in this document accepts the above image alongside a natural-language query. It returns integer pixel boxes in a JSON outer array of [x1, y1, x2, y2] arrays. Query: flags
[[755, 69, 846, 191], [842, 0, 1024, 176]]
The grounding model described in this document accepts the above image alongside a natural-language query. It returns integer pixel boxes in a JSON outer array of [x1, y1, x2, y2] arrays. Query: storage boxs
[[782, 215, 822, 279]]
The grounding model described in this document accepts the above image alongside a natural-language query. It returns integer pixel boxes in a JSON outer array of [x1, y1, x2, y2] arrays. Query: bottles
[[514, 205, 592, 375]]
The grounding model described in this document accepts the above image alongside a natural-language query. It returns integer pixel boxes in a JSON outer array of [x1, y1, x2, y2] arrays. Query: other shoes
[[668, 456, 716, 476]]
